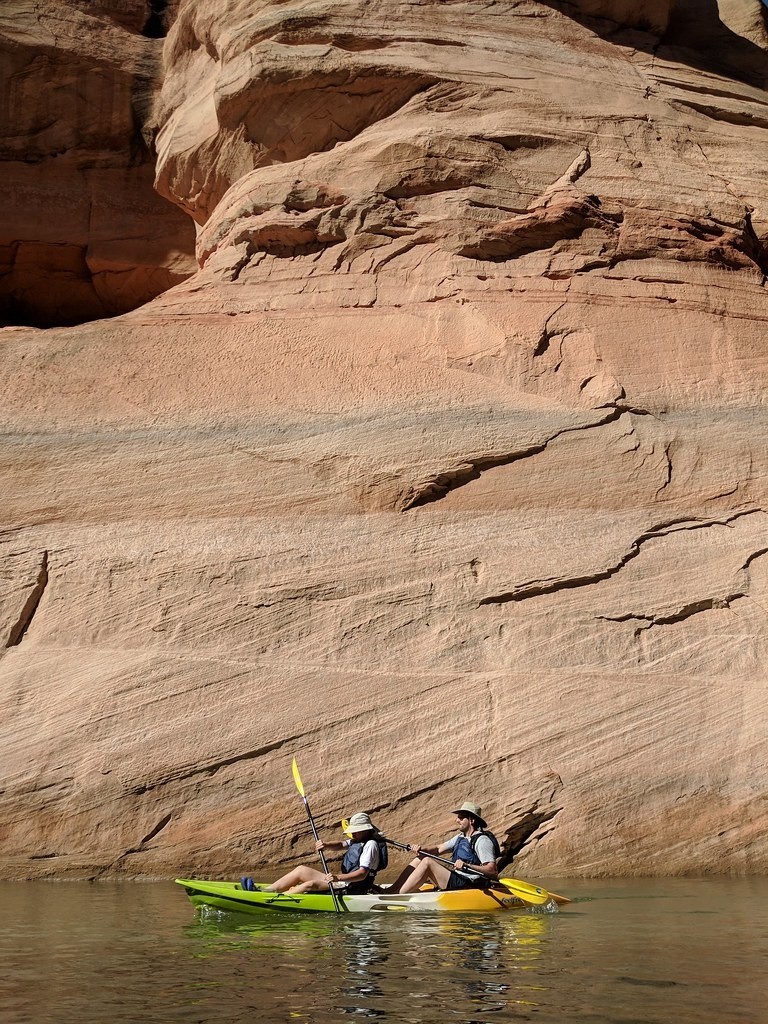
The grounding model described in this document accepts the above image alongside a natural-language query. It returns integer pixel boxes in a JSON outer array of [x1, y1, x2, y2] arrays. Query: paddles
[[341, 818, 550, 906], [289, 754, 341, 914]]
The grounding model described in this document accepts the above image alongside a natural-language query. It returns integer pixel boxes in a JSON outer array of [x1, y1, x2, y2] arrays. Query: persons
[[240, 813, 379, 894], [386, 801, 498, 894]]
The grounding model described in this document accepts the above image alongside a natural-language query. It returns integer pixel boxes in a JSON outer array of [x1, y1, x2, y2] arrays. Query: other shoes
[[242, 877, 261, 892], [371, 883, 385, 892]]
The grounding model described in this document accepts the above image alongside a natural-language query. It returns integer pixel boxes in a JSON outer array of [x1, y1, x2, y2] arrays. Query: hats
[[451, 801, 488, 828], [342, 813, 374, 835]]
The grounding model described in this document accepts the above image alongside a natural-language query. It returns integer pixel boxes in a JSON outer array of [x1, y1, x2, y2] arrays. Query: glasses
[[458, 815, 472, 821]]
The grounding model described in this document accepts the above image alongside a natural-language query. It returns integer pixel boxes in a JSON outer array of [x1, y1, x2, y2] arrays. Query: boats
[[175, 878, 573, 918]]
